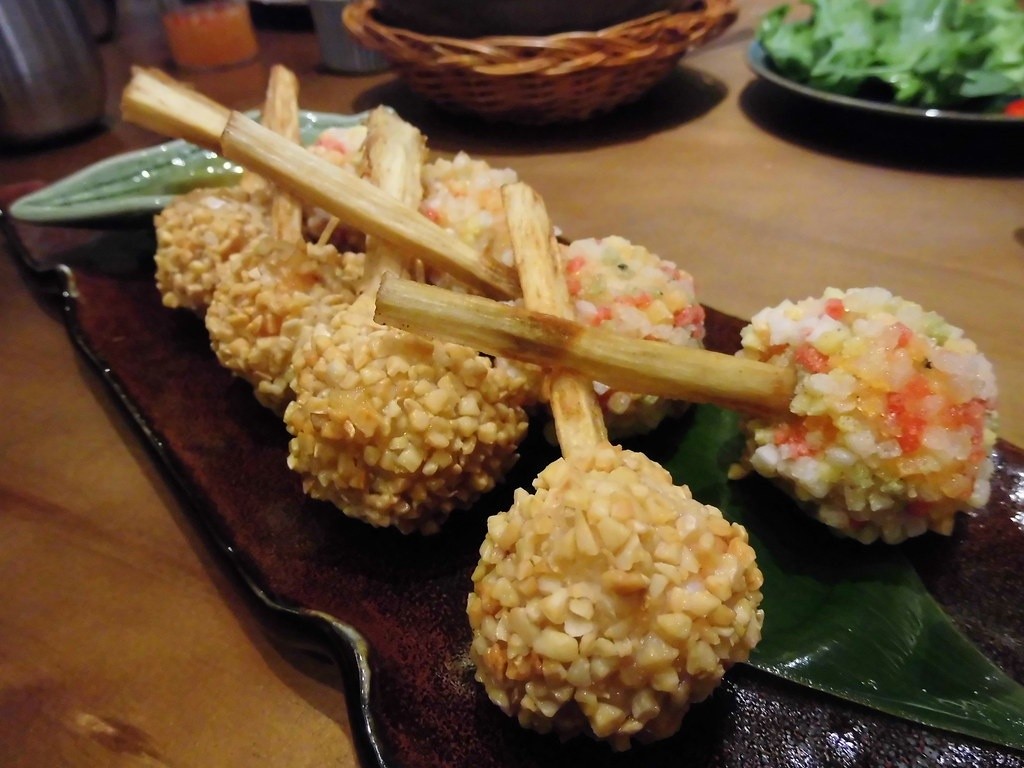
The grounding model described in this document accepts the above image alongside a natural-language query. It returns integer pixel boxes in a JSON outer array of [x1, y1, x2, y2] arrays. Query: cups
[[156, 0, 258, 71], [309, 0, 387, 73]]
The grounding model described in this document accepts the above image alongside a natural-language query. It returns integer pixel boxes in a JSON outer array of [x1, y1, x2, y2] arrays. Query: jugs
[[0, 0, 119, 142]]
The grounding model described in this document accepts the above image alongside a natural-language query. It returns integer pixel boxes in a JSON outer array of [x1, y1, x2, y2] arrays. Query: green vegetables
[[753, 1, 1023, 111]]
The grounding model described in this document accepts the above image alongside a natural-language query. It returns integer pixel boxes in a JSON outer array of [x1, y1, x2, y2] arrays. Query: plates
[[745, 41, 1024, 124], [3, 176, 1024, 768], [8, 108, 372, 230]]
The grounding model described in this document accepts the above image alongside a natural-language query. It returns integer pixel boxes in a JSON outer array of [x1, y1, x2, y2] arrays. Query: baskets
[[343, 1, 739, 123]]
